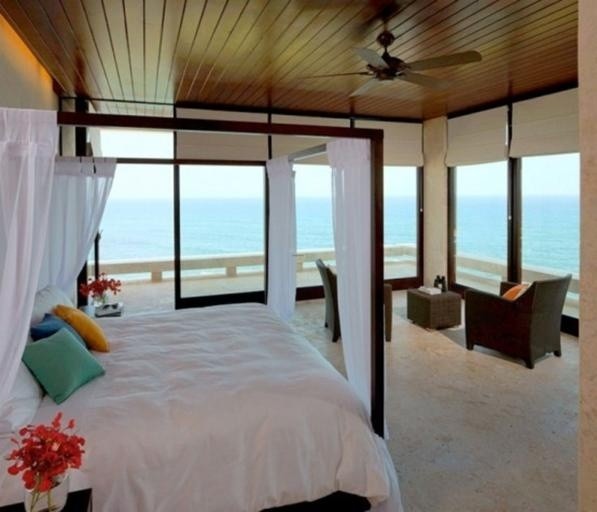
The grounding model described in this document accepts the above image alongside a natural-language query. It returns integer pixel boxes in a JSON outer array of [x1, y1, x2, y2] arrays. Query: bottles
[[434, 275, 446, 292]]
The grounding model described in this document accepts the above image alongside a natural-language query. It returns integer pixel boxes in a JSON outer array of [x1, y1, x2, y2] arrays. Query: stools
[[406, 286, 461, 331]]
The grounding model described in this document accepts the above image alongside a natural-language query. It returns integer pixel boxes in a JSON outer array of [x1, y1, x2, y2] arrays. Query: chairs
[[313, 257, 394, 345], [462, 272, 572, 370]]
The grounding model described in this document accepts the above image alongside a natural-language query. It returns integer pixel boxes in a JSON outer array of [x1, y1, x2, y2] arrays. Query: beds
[[0, 107, 404, 512]]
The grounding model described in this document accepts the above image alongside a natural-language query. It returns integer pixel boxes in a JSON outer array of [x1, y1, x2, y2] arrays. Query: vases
[[18, 470, 71, 511], [91, 293, 109, 308]]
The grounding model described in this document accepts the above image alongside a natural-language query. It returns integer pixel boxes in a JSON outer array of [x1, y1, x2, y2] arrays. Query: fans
[[298, 19, 488, 101]]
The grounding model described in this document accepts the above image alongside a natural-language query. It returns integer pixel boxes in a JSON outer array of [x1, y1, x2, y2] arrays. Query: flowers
[[77, 272, 122, 303], [0, 412, 88, 511]]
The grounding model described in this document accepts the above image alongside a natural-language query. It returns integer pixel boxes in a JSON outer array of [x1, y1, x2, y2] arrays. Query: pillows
[[498, 282, 528, 300], [30, 312, 88, 353], [23, 326, 107, 404], [25, 283, 73, 331], [51, 305, 110, 355], [3, 357, 43, 430]]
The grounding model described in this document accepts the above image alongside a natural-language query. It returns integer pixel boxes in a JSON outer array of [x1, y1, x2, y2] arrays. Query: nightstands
[[1, 487, 96, 512], [78, 304, 125, 318]]
